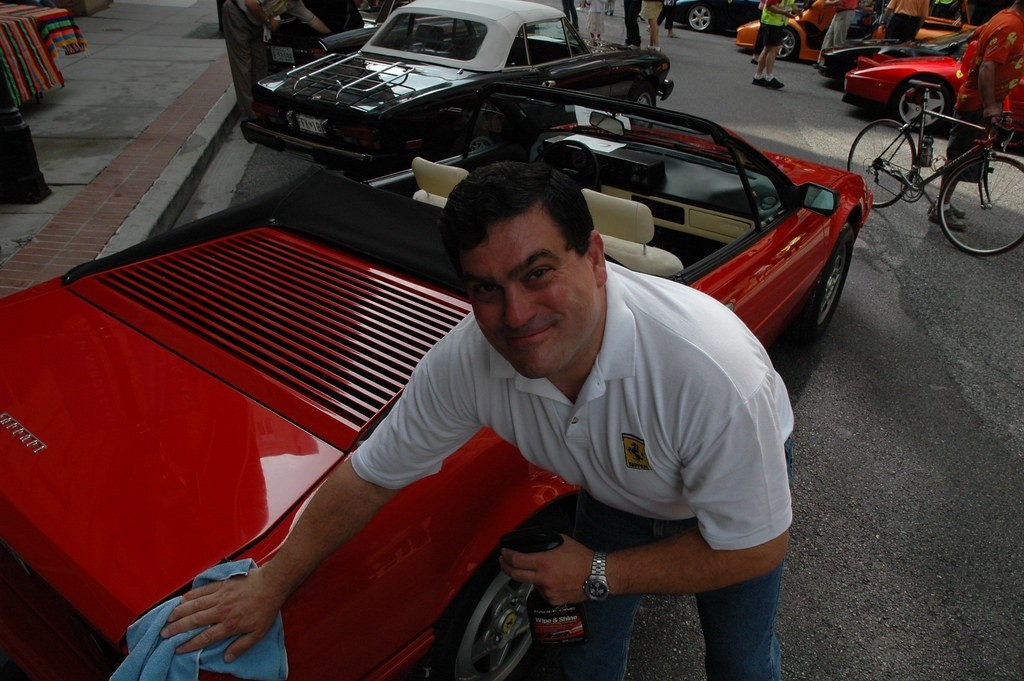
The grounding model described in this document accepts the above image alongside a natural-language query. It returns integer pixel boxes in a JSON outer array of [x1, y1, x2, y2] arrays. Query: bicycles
[[846, 80, 1024, 257]]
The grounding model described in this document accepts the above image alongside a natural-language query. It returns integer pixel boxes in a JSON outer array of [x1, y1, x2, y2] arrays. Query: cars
[[0, 80, 874, 681], [239, 0, 676, 171], [813, 30, 976, 80], [265, 1, 487, 69], [673, 0, 762, 34]]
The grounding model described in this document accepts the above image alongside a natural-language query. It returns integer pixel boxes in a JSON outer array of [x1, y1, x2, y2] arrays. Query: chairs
[[410, 155, 470, 207], [415, 24, 445, 57], [581, 188, 684, 277]]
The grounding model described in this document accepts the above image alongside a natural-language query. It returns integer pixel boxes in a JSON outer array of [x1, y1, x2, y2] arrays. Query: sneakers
[[752, 77, 784, 89], [927, 198, 966, 229]]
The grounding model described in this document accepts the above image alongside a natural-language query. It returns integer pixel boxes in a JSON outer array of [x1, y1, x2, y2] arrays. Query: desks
[[0, 2, 88, 105]]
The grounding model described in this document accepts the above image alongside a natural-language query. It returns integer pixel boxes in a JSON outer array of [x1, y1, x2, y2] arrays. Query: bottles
[[920, 132, 933, 168]]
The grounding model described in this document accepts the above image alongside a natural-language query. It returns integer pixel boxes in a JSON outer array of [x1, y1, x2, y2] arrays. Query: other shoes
[[605, 12, 613, 16], [590, 36, 601, 46], [667, 34, 678, 38], [622, 43, 641, 50], [647, 45, 661, 53], [750, 59, 758, 65]]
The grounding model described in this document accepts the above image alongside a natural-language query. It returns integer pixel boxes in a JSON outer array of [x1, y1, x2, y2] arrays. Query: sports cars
[[733, 0, 977, 62], [844, 52, 1024, 135]]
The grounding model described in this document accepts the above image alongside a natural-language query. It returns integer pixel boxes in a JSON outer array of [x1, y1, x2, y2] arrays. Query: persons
[[644, 0, 664, 53], [880, 0, 927, 44], [580, 0, 646, 22], [562, 0, 579, 33], [812, 0, 859, 68], [159, 160, 796, 681], [623, 0, 642, 50], [750, 0, 765, 64], [752, 0, 804, 89], [222, 0, 330, 124], [586, 0, 608, 46], [646, 0, 678, 39], [928, 0, 1024, 230]]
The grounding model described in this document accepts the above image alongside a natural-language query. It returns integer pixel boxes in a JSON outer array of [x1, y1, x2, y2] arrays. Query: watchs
[[583, 554, 610, 601]]
